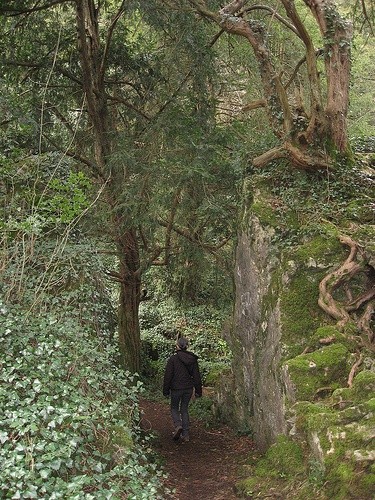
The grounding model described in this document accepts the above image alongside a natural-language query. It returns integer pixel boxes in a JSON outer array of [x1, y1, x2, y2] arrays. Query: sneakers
[[180, 434, 190, 442], [173, 426, 183, 441]]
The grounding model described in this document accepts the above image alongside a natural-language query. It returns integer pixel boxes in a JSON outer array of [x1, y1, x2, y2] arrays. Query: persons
[[163, 336, 202, 442]]
